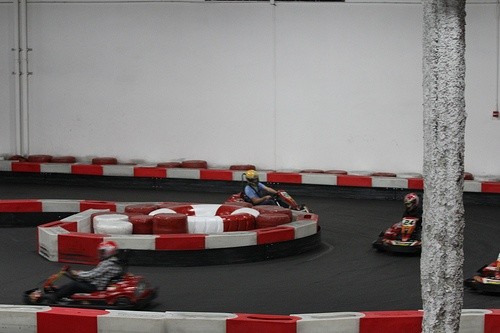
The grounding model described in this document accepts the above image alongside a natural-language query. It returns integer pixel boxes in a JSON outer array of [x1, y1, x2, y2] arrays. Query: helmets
[[404, 192, 419, 212], [246, 170, 259, 186], [98, 241, 119, 259]]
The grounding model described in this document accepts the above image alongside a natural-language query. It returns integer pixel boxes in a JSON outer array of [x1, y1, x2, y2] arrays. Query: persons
[[244, 170, 290, 208], [402, 192, 423, 241], [48, 240, 127, 305]]
